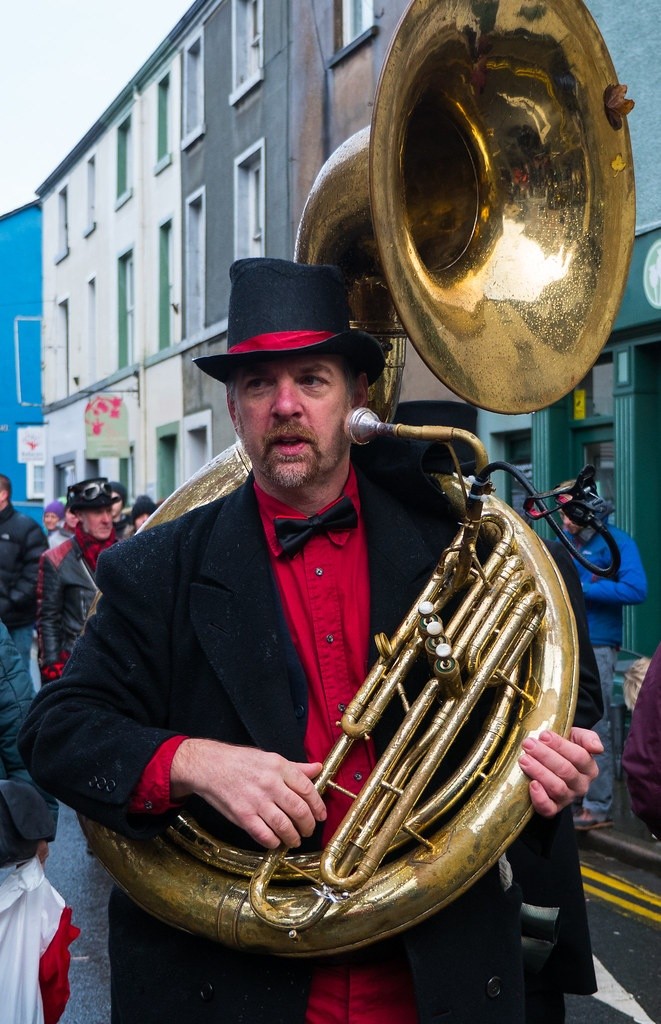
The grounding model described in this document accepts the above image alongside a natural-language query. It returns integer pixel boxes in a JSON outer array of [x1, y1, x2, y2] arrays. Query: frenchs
[[74, 0, 638, 958]]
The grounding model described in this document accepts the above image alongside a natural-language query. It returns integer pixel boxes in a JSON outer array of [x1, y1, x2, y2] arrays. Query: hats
[[70, 478, 120, 516], [105, 483, 127, 505], [192, 257, 386, 387], [45, 502, 67, 521], [131, 494, 158, 522]]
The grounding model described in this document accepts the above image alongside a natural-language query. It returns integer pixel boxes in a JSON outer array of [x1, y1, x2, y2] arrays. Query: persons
[[619, 642, 661, 841], [554, 478, 648, 831], [1, 475, 165, 1024], [18, 255, 604, 1024]]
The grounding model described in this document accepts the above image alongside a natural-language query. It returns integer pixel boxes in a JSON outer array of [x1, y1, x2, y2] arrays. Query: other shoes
[[572, 807, 615, 829]]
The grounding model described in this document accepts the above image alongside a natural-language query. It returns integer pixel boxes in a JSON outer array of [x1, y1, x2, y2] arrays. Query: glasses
[[82, 482, 112, 500]]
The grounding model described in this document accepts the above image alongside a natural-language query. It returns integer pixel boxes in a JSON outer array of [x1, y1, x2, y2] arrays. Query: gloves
[[43, 662, 65, 682]]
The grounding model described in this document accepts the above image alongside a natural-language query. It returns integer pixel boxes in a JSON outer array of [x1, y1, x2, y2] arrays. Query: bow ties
[[273, 495, 358, 562]]
